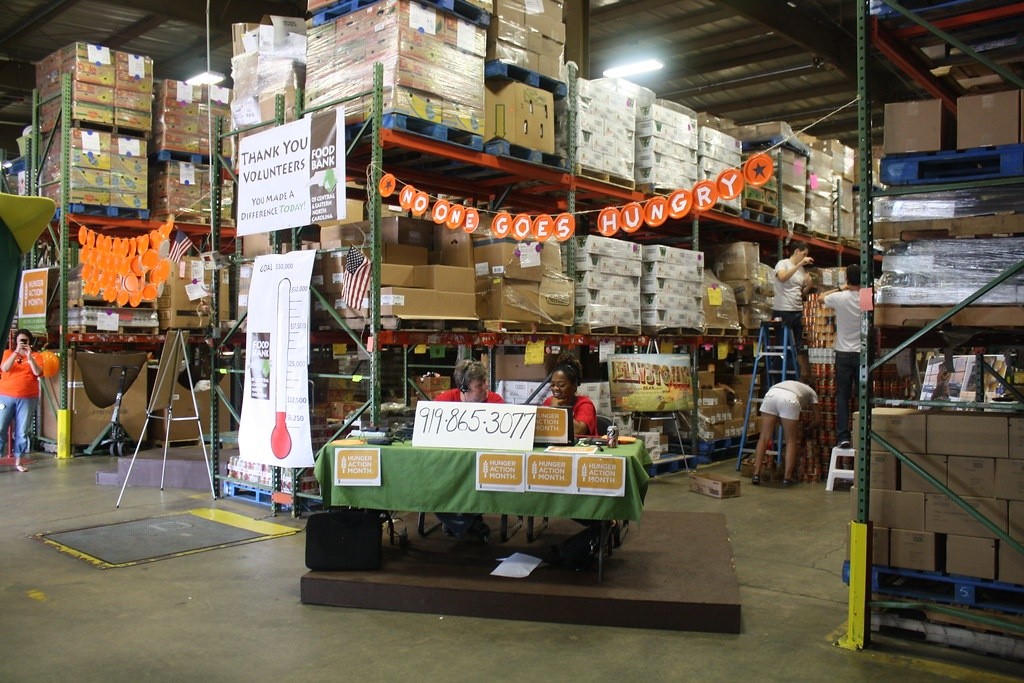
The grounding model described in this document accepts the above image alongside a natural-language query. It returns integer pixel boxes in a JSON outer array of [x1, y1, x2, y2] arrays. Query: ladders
[[736, 320, 802, 473]]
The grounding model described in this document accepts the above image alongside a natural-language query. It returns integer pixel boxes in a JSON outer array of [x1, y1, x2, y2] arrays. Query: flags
[[168, 230, 193, 264], [340, 246, 372, 310]]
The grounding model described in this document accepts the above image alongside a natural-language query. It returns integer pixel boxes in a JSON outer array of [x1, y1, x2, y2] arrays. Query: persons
[[932, 362, 950, 401], [771, 239, 814, 386], [818, 264, 862, 448], [543, 353, 621, 547], [752, 380, 820, 488], [431, 355, 505, 543], [966, 357, 997, 391], [0, 329, 43, 472]]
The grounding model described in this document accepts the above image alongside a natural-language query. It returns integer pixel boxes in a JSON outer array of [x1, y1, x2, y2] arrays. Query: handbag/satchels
[[305, 509, 383, 571]]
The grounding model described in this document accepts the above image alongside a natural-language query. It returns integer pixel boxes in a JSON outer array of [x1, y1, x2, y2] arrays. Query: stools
[[826, 445, 855, 491]]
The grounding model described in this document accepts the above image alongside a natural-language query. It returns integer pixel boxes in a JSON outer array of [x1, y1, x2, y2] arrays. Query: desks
[[313, 428, 653, 585]]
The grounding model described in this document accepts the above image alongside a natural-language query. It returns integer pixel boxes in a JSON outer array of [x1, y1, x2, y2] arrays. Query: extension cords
[[350, 430, 392, 438]]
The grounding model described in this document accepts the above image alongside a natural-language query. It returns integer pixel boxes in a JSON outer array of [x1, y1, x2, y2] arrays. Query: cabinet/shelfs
[[30, 72, 244, 459], [208, 60, 1024, 585], [0, 137, 29, 350], [833, 0, 1024, 655]]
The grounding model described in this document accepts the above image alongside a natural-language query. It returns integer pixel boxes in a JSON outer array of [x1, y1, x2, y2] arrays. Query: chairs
[[417, 512, 523, 543], [526, 415, 629, 546]]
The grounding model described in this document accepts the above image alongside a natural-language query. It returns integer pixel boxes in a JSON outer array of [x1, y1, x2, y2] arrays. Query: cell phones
[[21, 339, 27, 350]]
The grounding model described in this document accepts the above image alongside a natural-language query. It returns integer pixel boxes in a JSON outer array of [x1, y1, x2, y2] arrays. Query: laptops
[[533, 406, 579, 447]]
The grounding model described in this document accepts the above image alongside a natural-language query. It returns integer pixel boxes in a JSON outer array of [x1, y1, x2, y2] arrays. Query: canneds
[[607, 426, 618, 448]]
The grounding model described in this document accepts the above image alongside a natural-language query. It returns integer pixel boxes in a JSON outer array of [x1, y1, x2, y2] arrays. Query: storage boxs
[[1, 0, 855, 496]]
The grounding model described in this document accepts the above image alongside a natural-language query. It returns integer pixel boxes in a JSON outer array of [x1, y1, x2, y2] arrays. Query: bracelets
[[28, 357, 33, 363], [14, 351, 19, 354], [836, 287, 842, 291]]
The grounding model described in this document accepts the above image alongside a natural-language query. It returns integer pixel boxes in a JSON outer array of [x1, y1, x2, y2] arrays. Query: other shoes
[[481, 526, 491, 543], [752, 475, 759, 484], [783, 479, 796, 487], [16, 465, 28, 472], [838, 440, 850, 448], [442, 522, 451, 537]]
